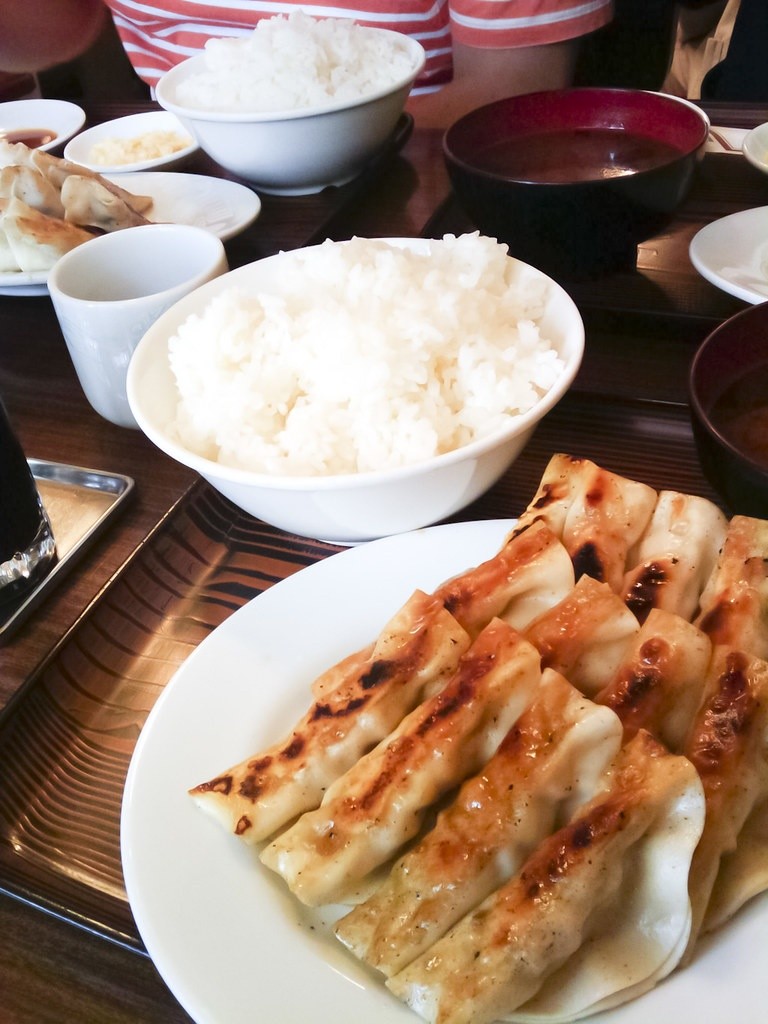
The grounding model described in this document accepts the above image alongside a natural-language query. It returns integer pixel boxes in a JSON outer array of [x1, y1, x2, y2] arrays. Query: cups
[[45, 222, 230, 428]]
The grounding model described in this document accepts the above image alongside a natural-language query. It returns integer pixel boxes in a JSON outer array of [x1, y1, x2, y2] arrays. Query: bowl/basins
[[127, 238, 585, 545], [689, 302, 768, 519], [442, 86, 711, 245], [153, 26, 430, 197]]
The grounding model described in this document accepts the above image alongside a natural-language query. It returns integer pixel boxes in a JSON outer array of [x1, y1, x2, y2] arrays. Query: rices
[[168, 229, 566, 479], [172, 9, 413, 112]]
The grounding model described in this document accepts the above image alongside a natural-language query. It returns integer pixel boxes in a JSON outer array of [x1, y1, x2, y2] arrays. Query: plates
[[743, 121, 768, 171], [64, 111, 203, 172], [0, 99, 87, 156], [0, 174, 262, 298], [689, 204, 768, 302], [119, 518, 768, 1024]]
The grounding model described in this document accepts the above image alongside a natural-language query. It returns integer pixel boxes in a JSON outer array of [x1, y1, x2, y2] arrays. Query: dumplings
[[189, 451, 767, 1024], [0, 141, 165, 272]]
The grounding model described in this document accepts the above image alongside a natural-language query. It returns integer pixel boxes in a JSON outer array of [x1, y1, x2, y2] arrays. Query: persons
[[0, 0, 613, 130]]
